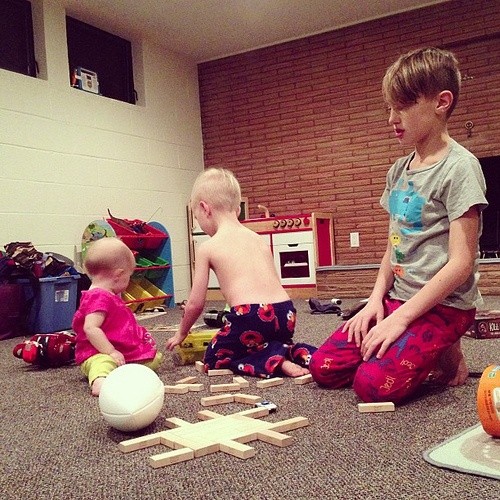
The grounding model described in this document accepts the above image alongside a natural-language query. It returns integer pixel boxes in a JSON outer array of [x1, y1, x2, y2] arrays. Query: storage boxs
[[108, 219, 169, 313], [465, 310, 500, 338], [10, 274, 82, 333]]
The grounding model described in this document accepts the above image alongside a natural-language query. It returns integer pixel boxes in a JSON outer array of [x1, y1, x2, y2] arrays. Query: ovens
[[273, 243, 316, 285]]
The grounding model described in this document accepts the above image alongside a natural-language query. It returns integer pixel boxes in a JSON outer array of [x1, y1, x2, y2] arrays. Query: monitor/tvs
[[477, 155, 500, 258]]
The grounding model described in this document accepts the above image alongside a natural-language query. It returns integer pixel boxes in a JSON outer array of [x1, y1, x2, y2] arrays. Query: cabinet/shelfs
[[79, 218, 175, 310], [189, 195, 335, 290]]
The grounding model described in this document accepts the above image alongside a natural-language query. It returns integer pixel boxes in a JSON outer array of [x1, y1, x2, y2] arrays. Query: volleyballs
[[95, 362, 169, 432]]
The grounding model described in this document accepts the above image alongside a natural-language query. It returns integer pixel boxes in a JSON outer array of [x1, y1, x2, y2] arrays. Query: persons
[[163, 167, 319, 378], [309, 45, 489, 407], [71, 238, 163, 395]]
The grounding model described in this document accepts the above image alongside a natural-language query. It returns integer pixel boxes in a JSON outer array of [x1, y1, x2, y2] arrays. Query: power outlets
[[350, 232, 359, 247]]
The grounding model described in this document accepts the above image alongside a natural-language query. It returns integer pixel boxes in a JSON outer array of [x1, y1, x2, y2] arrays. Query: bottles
[[330, 299, 341, 305]]
